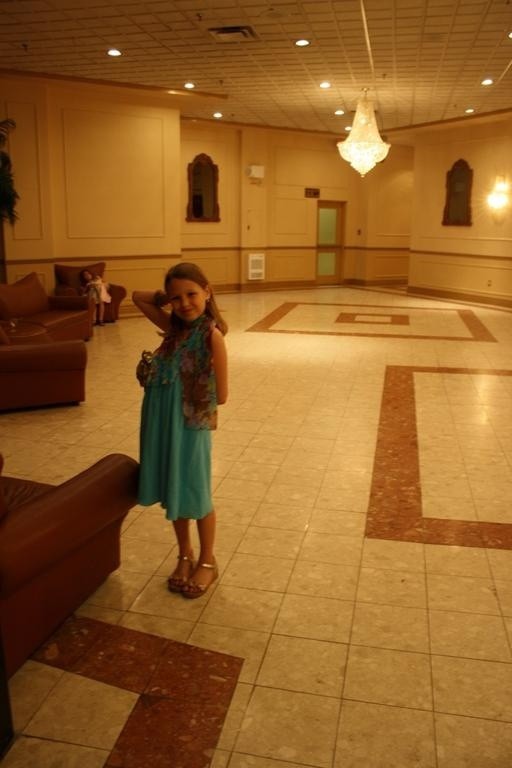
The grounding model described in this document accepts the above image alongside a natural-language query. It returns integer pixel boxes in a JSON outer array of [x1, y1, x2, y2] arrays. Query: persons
[[130, 259, 231, 600], [79, 268, 113, 326]]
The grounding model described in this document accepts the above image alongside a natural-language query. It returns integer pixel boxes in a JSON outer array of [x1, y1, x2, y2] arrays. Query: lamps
[[337, 87, 391, 180], [489, 175, 508, 223]]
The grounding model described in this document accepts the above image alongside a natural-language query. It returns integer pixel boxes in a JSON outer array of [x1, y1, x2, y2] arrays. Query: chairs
[[1, 452, 142, 681], [0, 272, 94, 342], [0, 339, 87, 413], [53, 261, 126, 325]]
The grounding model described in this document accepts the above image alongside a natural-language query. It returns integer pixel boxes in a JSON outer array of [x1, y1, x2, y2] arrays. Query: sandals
[[167, 553, 194, 593], [183, 557, 220, 599]]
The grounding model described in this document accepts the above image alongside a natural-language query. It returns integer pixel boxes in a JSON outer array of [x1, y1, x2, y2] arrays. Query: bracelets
[[152, 289, 168, 308]]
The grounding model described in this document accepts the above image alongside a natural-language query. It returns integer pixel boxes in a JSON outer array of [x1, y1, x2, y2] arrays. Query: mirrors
[[185, 153, 220, 222]]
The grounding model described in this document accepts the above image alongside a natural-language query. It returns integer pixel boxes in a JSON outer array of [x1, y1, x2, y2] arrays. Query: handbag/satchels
[[136, 350, 153, 388]]
